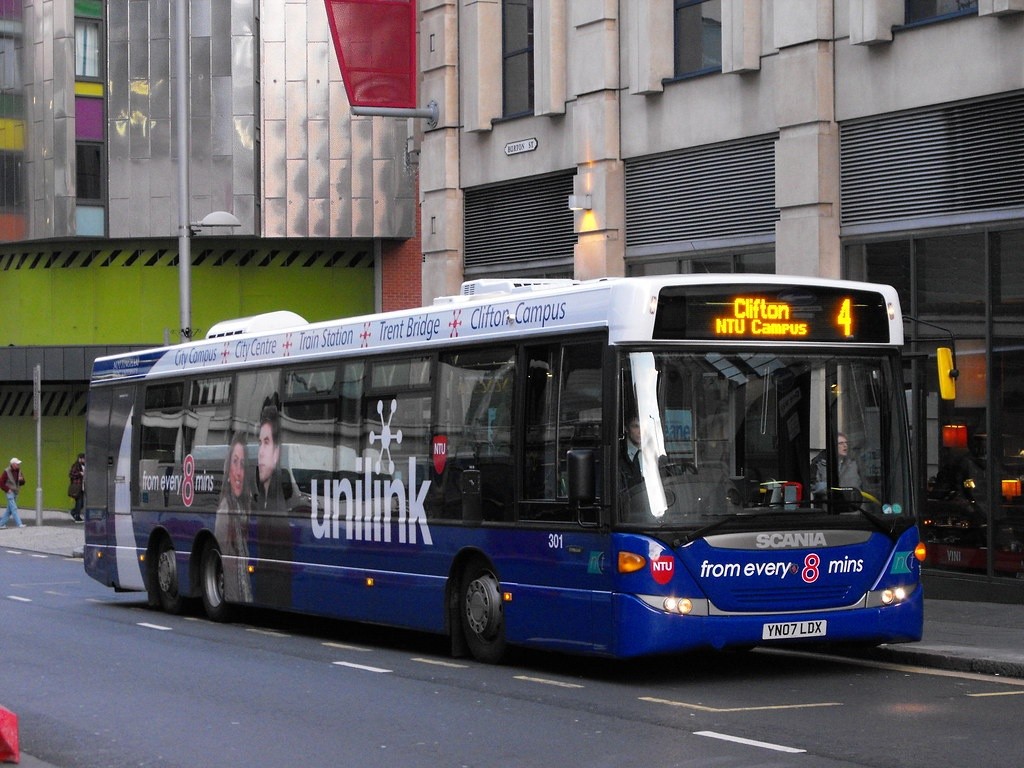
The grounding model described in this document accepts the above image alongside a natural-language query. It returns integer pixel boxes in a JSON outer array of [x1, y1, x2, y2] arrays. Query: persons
[[253, 404, 292, 608], [619, 408, 656, 488], [68, 452, 85, 524], [814, 433, 862, 501], [0, 458, 26, 529], [212, 436, 254, 602]]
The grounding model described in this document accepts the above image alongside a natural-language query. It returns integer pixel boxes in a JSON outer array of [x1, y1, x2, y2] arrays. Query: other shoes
[[70, 511, 76, 521], [0, 524, 8, 529], [18, 523, 27, 527], [75, 517, 84, 524]]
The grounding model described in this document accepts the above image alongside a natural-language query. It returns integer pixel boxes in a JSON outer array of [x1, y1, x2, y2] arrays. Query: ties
[[632, 448, 641, 479]]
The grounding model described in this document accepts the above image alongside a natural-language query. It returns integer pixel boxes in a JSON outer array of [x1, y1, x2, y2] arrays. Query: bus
[[82, 277, 959, 673]]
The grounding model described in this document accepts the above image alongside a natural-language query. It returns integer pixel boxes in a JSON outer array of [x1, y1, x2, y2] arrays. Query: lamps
[[1001, 479, 1022, 504], [568, 194, 592, 211]]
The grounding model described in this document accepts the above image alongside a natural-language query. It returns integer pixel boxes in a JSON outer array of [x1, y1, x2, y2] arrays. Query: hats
[[10, 458, 22, 464]]
